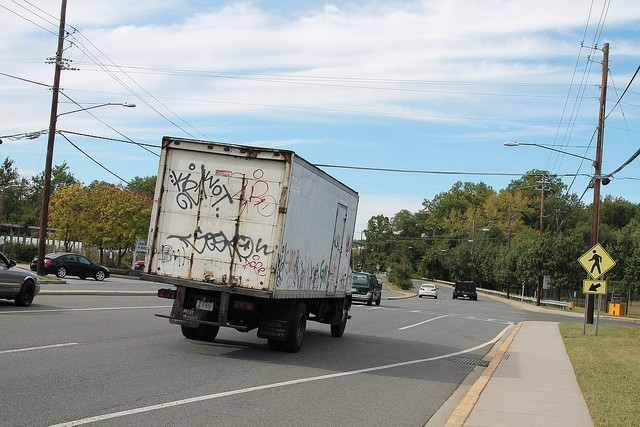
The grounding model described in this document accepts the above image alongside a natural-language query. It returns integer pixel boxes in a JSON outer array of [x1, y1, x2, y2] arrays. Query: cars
[[377, 278, 383, 287], [30, 252, 110, 281], [0, 251, 40, 307], [418, 284, 438, 299]]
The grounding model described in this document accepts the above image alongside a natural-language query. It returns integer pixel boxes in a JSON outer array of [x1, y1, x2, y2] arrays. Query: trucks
[[141, 135, 362, 353]]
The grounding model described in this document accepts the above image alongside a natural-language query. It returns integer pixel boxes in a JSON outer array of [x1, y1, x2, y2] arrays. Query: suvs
[[351, 271, 382, 306], [453, 279, 477, 300]]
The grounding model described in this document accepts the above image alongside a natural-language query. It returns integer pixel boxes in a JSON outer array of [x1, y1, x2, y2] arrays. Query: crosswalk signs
[[577, 242, 617, 295]]
